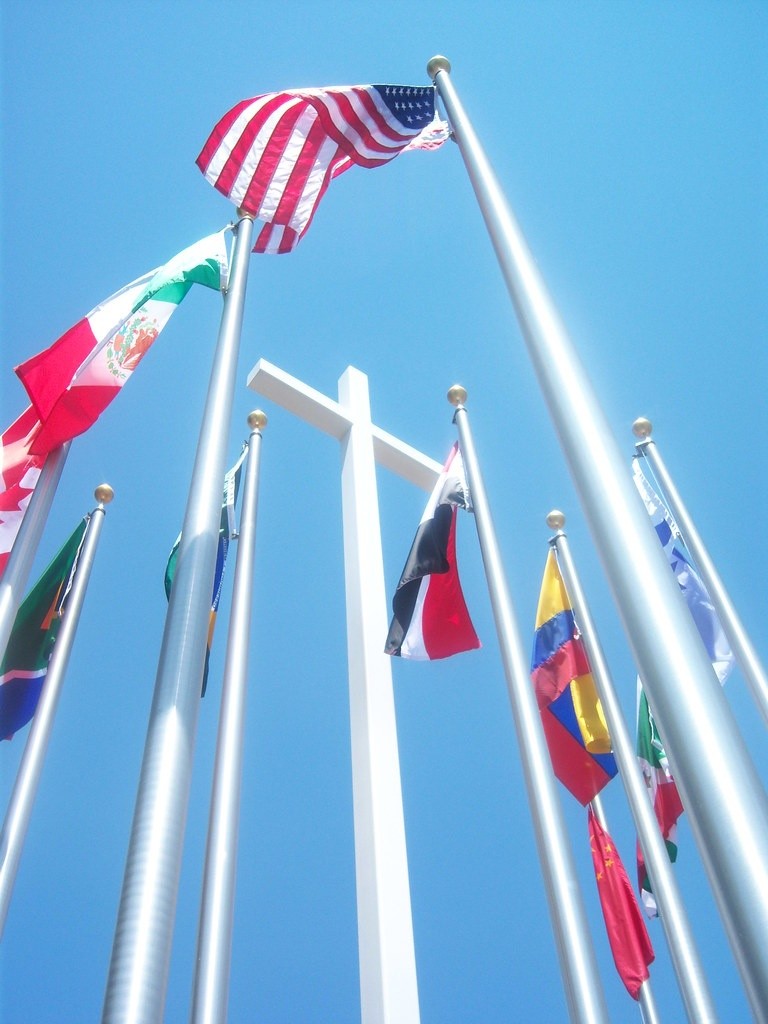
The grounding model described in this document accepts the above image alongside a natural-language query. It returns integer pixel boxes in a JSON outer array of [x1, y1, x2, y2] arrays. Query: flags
[[1, 404, 49, 579], [0, 513, 90, 742], [633, 674, 684, 919], [164, 447, 249, 698], [633, 461, 734, 684], [531, 542, 618, 806], [384, 441, 483, 660], [588, 804, 655, 999], [196, 82, 450, 255], [15, 231, 228, 455]]
